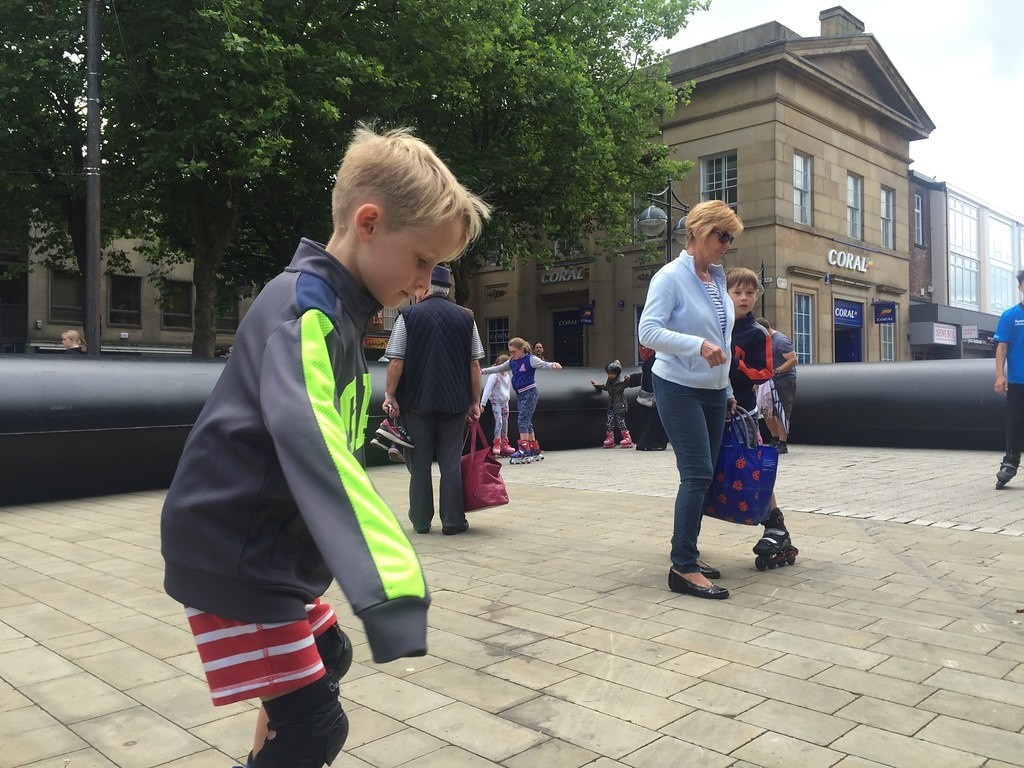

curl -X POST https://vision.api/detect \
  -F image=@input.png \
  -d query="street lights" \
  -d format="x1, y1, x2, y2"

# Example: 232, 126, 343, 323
637, 176, 690, 263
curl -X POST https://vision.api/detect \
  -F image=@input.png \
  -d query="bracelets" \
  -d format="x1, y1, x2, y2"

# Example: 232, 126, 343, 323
385, 393, 396, 398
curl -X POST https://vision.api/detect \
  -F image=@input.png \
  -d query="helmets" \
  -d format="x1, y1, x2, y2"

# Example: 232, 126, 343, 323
605, 362, 622, 377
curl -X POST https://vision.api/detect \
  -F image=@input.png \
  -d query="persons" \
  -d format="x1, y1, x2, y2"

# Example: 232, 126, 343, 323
993, 270, 1024, 490
220, 345, 233, 359
533, 342, 545, 361
638, 199, 743, 598
480, 338, 562, 464
591, 346, 667, 450
382, 266, 485, 535
480, 354, 515, 458
757, 318, 797, 454
62, 330, 87, 354
161, 127, 484, 768
726, 267, 799, 571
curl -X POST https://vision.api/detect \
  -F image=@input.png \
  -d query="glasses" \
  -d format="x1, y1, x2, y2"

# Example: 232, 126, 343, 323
534, 346, 543, 350
712, 229, 735, 246
509, 348, 521, 356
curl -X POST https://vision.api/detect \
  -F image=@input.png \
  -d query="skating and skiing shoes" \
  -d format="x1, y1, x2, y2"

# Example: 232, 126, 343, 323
499, 436, 516, 457
996, 454, 1021, 488
509, 438, 532, 464
491, 436, 502, 459
528, 440, 544, 461
752, 509, 799, 571
619, 429, 633, 448
603, 430, 616, 448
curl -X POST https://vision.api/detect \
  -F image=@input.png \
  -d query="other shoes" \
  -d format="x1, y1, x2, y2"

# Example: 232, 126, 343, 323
648, 446, 664, 450
774, 443, 789, 454
667, 567, 730, 600
416, 526, 430, 533
442, 517, 468, 534
695, 559, 720, 579
768, 438, 780, 448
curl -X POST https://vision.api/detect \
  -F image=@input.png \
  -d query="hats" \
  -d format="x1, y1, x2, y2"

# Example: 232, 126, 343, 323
431, 265, 453, 289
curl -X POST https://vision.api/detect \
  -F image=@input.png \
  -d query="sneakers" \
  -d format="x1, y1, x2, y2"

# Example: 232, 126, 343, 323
376, 416, 415, 449
370, 435, 392, 452
387, 441, 406, 463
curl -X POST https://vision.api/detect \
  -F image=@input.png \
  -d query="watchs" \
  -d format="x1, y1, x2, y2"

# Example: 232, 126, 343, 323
774, 369, 778, 375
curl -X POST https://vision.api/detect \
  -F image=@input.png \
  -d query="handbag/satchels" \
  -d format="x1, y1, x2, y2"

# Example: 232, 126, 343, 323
636, 389, 656, 407
460, 419, 509, 513
701, 404, 779, 525
